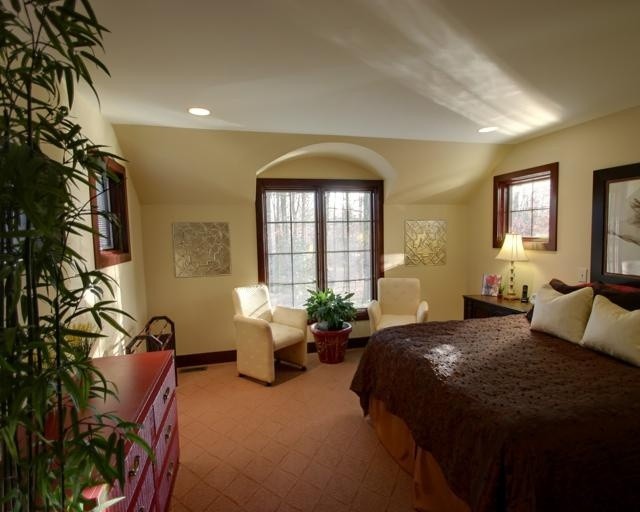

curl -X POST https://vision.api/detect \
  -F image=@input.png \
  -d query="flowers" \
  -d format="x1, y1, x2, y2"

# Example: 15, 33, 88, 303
66, 323, 101, 359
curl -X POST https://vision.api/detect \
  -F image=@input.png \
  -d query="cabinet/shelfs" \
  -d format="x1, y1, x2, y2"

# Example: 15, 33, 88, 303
124, 316, 176, 391
0, 348, 180, 512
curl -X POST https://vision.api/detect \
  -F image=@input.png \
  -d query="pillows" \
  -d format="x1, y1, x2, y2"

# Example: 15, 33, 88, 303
531, 283, 595, 344
596, 289, 640, 311
582, 295, 640, 368
525, 279, 582, 320
577, 283, 638, 292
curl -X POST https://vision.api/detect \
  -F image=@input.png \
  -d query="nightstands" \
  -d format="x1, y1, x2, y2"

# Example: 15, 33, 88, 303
464, 295, 533, 319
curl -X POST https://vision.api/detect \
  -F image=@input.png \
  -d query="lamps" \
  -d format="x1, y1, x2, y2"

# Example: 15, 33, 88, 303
496, 234, 528, 300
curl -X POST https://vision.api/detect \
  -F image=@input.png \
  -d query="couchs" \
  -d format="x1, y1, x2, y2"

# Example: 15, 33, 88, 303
233, 284, 308, 385
367, 277, 428, 334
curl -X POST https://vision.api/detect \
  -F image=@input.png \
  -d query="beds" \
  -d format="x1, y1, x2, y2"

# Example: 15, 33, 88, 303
349, 278, 640, 512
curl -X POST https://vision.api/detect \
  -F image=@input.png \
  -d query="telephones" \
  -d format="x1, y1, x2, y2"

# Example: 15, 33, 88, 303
521, 285, 529, 303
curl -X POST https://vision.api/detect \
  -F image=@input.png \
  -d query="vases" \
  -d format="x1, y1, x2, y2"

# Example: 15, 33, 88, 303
81, 357, 95, 388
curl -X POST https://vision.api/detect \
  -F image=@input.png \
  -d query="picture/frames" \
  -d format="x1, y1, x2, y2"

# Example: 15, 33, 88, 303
590, 162, 640, 287
482, 273, 502, 297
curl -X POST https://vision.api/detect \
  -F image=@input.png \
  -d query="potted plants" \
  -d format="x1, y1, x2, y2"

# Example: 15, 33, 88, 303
303, 289, 357, 363
497, 283, 505, 298
